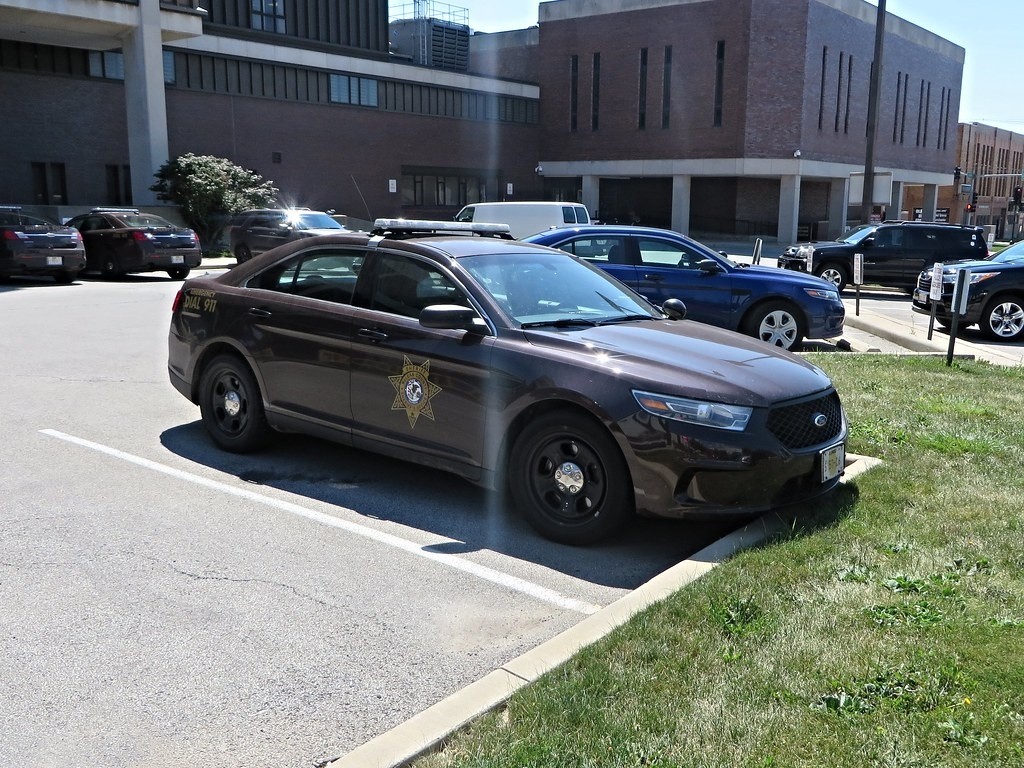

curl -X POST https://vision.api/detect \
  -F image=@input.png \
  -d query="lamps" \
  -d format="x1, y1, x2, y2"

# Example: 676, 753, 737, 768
506, 180, 513, 195
388, 177, 397, 193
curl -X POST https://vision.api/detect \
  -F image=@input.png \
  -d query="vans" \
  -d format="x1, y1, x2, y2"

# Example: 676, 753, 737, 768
450, 202, 594, 256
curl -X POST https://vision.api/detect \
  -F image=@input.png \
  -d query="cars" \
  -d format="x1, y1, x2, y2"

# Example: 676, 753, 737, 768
474, 225, 846, 353
0, 206, 87, 284
230, 210, 355, 270
911, 239, 1024, 344
168, 219, 849, 545
62, 208, 202, 282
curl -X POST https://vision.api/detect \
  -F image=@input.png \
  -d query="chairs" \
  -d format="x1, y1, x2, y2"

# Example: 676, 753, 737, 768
99, 217, 118, 229
7, 215, 29, 225
606, 243, 626, 265
878, 229, 892, 245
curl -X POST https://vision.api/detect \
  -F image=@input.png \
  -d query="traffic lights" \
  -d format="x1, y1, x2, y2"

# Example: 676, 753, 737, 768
1013, 187, 1022, 205
953, 166, 961, 180
967, 204, 971, 209
971, 192, 978, 212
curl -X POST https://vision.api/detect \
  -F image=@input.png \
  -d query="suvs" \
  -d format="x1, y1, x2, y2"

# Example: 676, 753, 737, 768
778, 220, 990, 294
